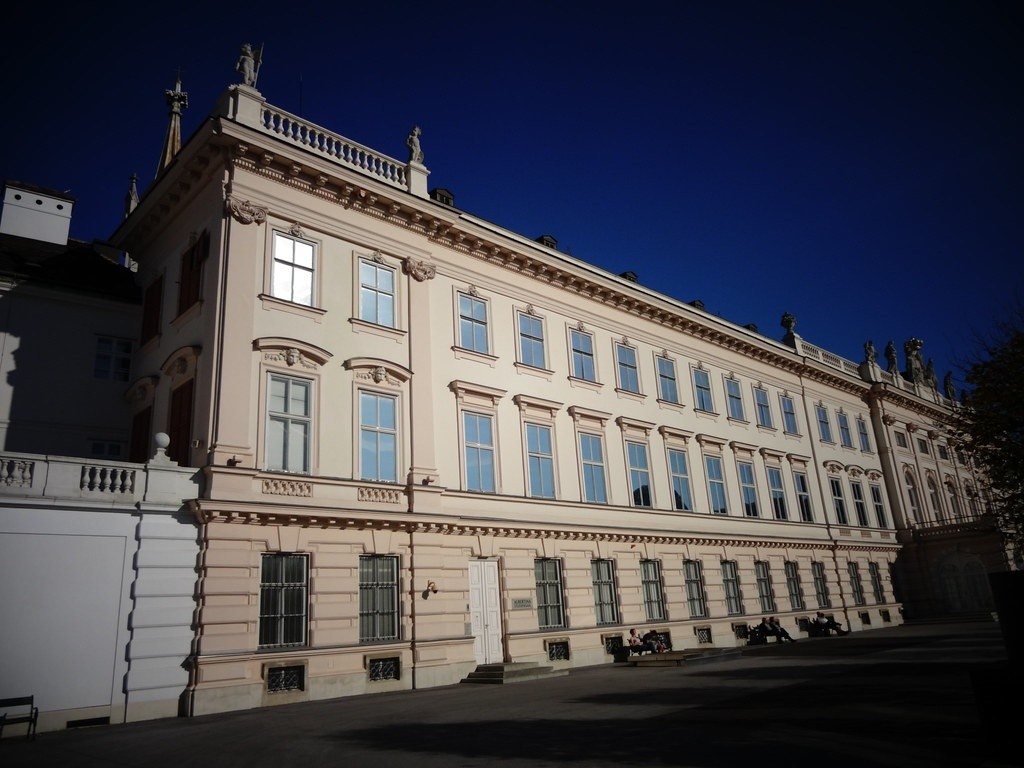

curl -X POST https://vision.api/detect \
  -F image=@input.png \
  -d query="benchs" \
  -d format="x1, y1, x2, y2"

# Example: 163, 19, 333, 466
627, 637, 663, 656
0, 695, 39, 740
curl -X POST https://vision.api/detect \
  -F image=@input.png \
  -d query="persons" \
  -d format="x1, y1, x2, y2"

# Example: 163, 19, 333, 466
816, 612, 849, 636
407, 128, 424, 163
759, 617, 797, 644
235, 41, 254, 84
884, 340, 897, 373
630, 629, 663, 653
864, 340, 876, 361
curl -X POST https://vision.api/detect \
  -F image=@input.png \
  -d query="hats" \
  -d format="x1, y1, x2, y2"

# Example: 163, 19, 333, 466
650, 629, 657, 636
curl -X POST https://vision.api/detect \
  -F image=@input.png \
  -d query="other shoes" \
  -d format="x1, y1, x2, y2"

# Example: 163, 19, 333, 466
779, 641, 784, 644
790, 640, 796, 643
